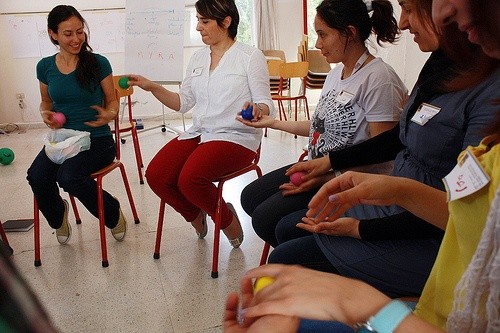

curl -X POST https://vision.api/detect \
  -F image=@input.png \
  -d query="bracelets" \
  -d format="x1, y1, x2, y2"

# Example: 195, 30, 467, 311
108, 108, 117, 120
356, 298, 411, 333
333, 169, 342, 177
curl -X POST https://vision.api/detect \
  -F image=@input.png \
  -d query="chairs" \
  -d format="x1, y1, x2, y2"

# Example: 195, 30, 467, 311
254, 144, 308, 286
113, 76, 144, 186
262, 39, 332, 139
33, 89, 140, 266
153, 143, 262, 278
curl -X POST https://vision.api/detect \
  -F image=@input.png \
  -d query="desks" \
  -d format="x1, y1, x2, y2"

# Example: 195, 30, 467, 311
120, 80, 187, 145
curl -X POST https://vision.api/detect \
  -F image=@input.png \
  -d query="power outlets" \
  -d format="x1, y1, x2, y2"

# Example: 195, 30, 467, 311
15, 92, 25, 99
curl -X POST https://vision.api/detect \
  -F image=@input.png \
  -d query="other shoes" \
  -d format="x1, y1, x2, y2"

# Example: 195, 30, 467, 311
195, 208, 209, 240
223, 202, 244, 249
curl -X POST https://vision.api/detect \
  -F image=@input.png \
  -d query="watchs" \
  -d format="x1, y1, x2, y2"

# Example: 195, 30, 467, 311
254, 103, 263, 110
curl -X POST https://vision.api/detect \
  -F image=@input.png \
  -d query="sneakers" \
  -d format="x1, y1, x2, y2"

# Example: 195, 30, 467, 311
111, 196, 127, 243
56, 199, 72, 245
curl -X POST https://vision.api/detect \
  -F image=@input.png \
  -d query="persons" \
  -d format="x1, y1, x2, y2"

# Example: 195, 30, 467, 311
224, 0, 500, 333
26, 4, 126, 245
121, 0, 275, 248
